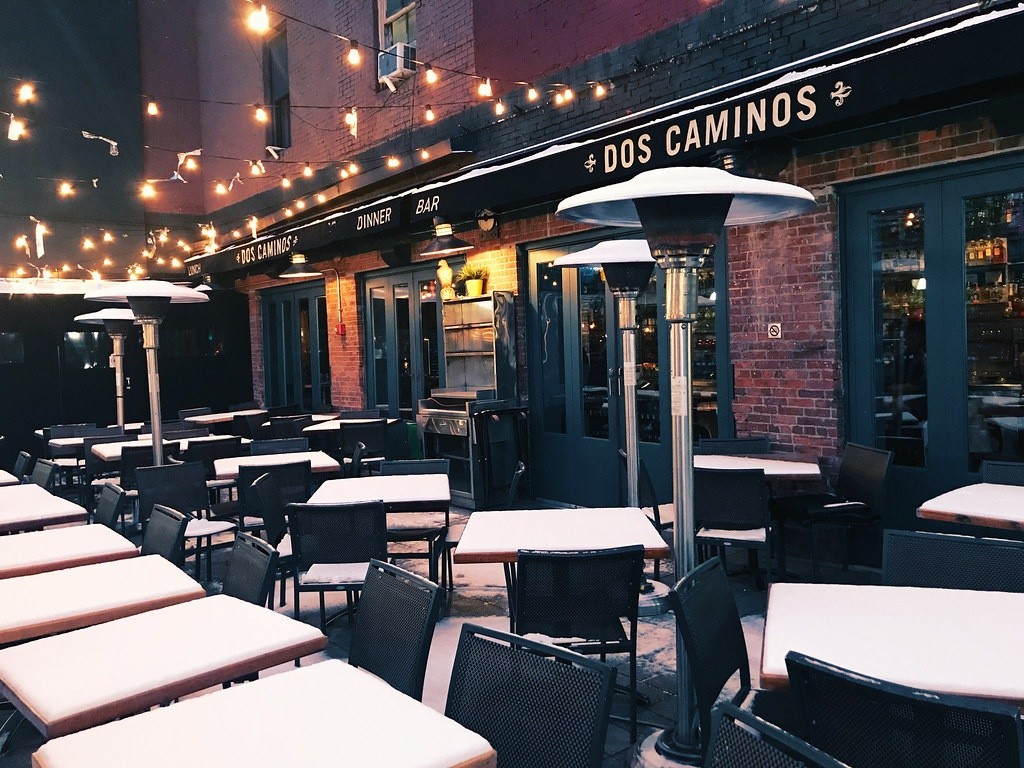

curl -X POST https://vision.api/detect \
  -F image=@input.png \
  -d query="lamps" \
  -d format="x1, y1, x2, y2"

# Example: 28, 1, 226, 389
278, 253, 322, 278
419, 216, 475, 256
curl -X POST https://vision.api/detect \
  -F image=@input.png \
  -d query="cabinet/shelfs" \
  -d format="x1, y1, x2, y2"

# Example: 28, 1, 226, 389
417, 289, 517, 513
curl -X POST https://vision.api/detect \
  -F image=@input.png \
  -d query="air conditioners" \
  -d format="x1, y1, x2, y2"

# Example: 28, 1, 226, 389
377, 41, 417, 85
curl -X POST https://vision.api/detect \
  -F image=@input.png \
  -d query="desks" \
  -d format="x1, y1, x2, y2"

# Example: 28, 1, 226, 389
0, 408, 1024, 768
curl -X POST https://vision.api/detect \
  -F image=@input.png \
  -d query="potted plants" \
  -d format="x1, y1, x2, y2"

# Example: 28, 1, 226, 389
455, 263, 492, 297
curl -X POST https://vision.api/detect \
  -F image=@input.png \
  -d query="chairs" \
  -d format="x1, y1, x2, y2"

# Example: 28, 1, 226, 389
11, 400, 1024, 768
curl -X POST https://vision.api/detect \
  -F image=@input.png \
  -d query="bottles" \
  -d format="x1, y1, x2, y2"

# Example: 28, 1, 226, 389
966, 277, 1024, 305
881, 248, 925, 272
966, 237, 1004, 263
